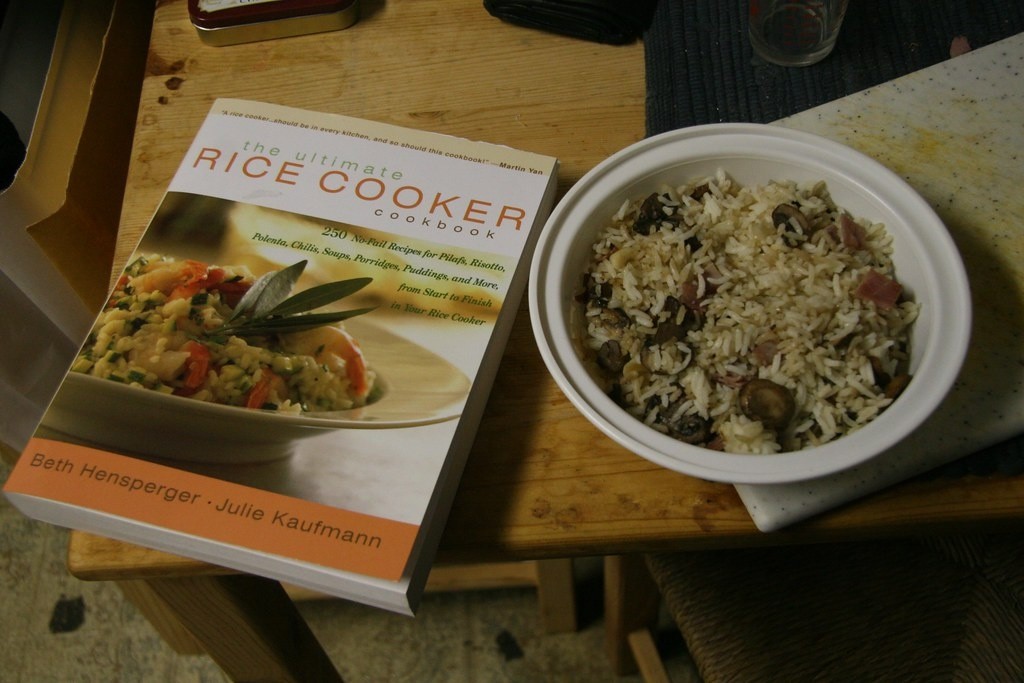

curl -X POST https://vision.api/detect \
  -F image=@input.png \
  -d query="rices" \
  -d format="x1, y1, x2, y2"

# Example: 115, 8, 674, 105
571, 166, 921, 453
70, 254, 378, 416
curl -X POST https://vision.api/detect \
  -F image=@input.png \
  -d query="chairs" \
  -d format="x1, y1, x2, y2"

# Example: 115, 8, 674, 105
606, 513, 1023, 683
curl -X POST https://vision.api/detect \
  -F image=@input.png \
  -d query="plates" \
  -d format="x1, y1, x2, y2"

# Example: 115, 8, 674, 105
40, 297, 473, 464
528, 124, 972, 483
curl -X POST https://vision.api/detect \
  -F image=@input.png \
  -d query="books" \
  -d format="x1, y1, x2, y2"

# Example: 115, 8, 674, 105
1, 98, 561, 618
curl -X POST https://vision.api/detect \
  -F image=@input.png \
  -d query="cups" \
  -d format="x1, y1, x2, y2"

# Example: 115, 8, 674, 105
747, 0, 848, 66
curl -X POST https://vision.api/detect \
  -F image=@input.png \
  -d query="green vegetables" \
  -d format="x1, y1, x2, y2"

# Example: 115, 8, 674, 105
206, 259, 380, 339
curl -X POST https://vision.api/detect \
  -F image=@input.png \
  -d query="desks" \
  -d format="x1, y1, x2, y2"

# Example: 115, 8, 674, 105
68, 0, 1023, 682
